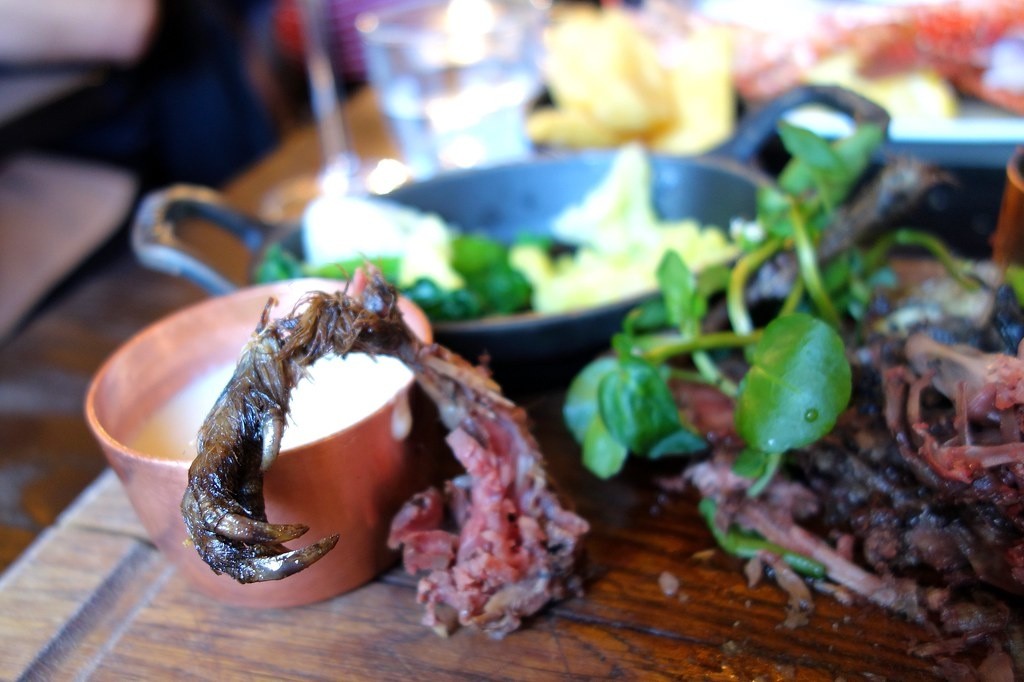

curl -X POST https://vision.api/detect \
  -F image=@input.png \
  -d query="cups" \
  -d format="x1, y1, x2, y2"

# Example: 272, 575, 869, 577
354, 0, 559, 185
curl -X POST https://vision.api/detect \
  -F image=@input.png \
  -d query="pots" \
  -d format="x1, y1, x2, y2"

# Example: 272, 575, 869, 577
126, 77, 892, 445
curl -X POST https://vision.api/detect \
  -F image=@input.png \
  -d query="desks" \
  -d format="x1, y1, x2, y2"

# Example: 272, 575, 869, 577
0, 256, 1024, 682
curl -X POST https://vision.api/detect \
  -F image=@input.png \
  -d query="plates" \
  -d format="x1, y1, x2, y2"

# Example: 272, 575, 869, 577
778, 42, 1024, 175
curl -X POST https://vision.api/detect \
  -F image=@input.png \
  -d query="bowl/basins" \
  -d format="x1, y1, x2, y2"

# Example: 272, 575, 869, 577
88, 275, 433, 612
